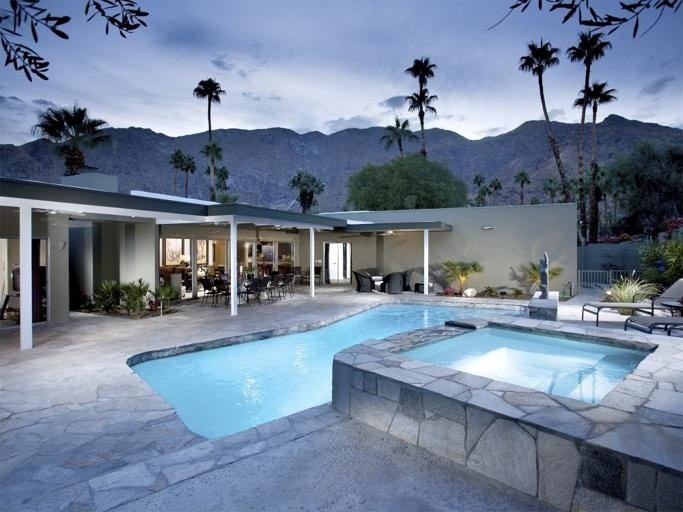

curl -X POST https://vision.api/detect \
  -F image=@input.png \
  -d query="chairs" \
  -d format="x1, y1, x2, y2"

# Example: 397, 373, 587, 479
582, 278, 683, 327
380, 272, 404, 294
201, 266, 321, 308
624, 315, 683, 336
353, 270, 372, 292
